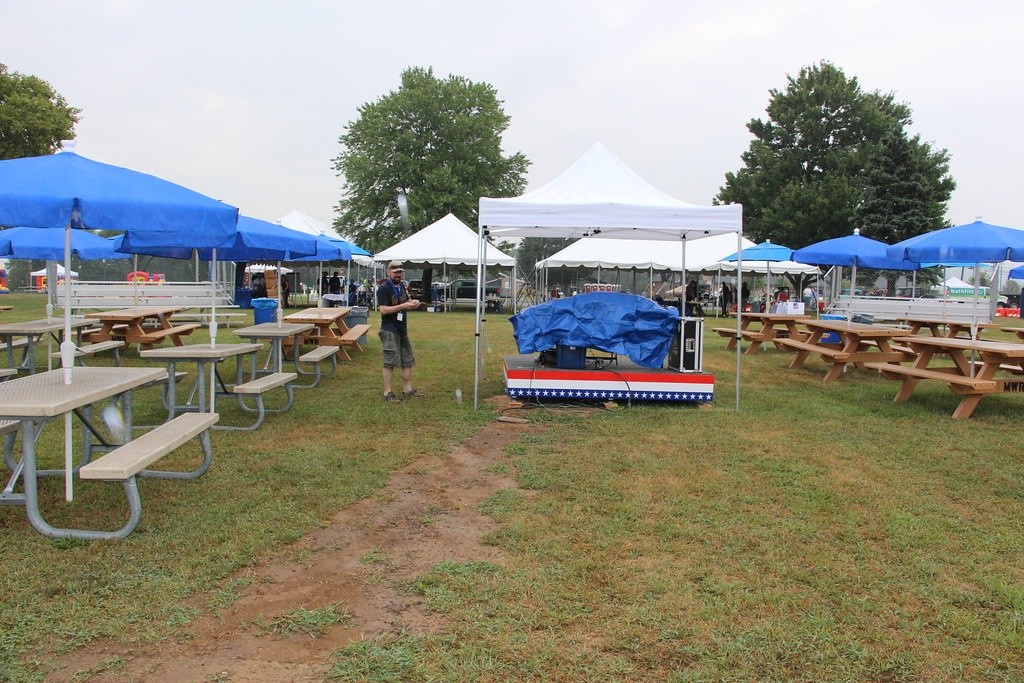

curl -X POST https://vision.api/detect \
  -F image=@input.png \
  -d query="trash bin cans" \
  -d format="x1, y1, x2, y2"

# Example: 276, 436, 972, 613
251, 298, 279, 325
322, 294, 344, 308
851, 313, 875, 325
345, 307, 369, 345
819, 313, 848, 343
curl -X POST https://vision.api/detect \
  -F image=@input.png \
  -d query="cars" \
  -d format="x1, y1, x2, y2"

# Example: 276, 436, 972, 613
326, 275, 501, 302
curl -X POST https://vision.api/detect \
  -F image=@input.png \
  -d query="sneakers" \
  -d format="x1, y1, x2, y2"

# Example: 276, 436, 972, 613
402, 389, 426, 399
383, 391, 400, 403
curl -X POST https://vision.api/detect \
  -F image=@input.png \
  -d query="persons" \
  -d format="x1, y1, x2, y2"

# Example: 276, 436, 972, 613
774, 285, 817, 310
254, 273, 268, 299
653, 294, 665, 305
861, 285, 910, 302
422, 260, 433, 304
494, 288, 505, 313
300, 282, 308, 294
281, 274, 290, 308
547, 288, 582, 300
317, 272, 408, 310
376, 261, 421, 401
685, 280, 750, 317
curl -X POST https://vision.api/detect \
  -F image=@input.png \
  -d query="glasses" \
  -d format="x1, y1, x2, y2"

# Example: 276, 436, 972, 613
389, 265, 403, 269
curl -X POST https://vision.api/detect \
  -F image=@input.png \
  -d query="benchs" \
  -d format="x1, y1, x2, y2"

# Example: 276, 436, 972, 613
0, 324, 373, 539
825, 294, 993, 331
711, 327, 1015, 390
55, 281, 247, 328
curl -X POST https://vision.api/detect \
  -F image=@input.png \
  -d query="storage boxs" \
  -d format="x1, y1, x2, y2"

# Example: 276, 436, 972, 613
264, 269, 285, 308
667, 317, 704, 373
556, 345, 586, 369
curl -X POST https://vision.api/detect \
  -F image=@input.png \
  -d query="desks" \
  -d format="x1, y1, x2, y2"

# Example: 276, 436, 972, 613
0, 305, 363, 540
725, 313, 1024, 420
485, 295, 504, 313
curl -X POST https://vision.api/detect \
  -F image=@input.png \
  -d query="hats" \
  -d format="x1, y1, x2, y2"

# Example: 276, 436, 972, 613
387, 261, 405, 272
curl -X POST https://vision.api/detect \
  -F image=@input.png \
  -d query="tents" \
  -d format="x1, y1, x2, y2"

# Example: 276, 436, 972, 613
930, 276, 986, 298
374, 213, 517, 314
532, 233, 822, 320
244, 211, 386, 283
474, 142, 743, 413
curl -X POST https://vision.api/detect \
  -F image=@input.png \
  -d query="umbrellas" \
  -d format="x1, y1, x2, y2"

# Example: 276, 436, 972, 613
790, 228, 921, 372
284, 234, 376, 335
717, 239, 793, 314
886, 216, 1024, 379
0, 151, 238, 504
0, 214, 317, 413
1007, 265, 1024, 279
